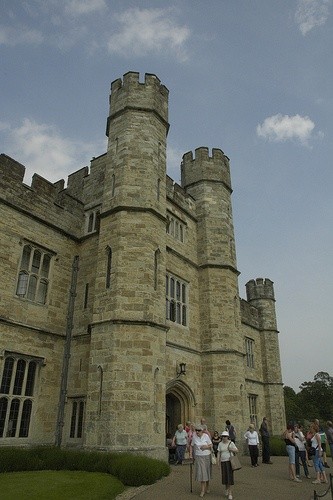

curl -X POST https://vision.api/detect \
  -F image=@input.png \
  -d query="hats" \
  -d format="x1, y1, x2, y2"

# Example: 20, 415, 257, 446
220, 430, 229, 436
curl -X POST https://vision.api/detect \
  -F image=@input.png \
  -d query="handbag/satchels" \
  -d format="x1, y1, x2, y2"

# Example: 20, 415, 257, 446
307, 444, 319, 456
228, 441, 241, 470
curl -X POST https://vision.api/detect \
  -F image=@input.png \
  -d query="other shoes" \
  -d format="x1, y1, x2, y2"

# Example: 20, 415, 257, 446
252, 463, 259, 467
289, 478, 302, 483
306, 475, 311, 478
311, 479, 328, 484
308, 464, 313, 467
296, 475, 300, 478
205, 488, 211, 493
200, 491, 205, 497
321, 462, 330, 468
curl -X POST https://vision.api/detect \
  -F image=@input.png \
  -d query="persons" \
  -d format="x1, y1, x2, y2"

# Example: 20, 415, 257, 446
166, 418, 211, 465
225, 419, 236, 444
259, 417, 273, 464
284, 418, 333, 485
211, 430, 222, 458
216, 430, 239, 500
188, 424, 214, 497
244, 424, 260, 468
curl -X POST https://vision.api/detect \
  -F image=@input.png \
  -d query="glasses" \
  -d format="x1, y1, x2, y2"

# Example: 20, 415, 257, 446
195, 429, 202, 432
221, 435, 228, 437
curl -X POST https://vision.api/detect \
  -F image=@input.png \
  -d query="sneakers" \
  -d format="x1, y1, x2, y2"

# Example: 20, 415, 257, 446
225, 490, 233, 499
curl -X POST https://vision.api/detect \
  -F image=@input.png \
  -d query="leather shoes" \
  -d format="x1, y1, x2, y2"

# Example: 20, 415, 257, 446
262, 461, 273, 464
